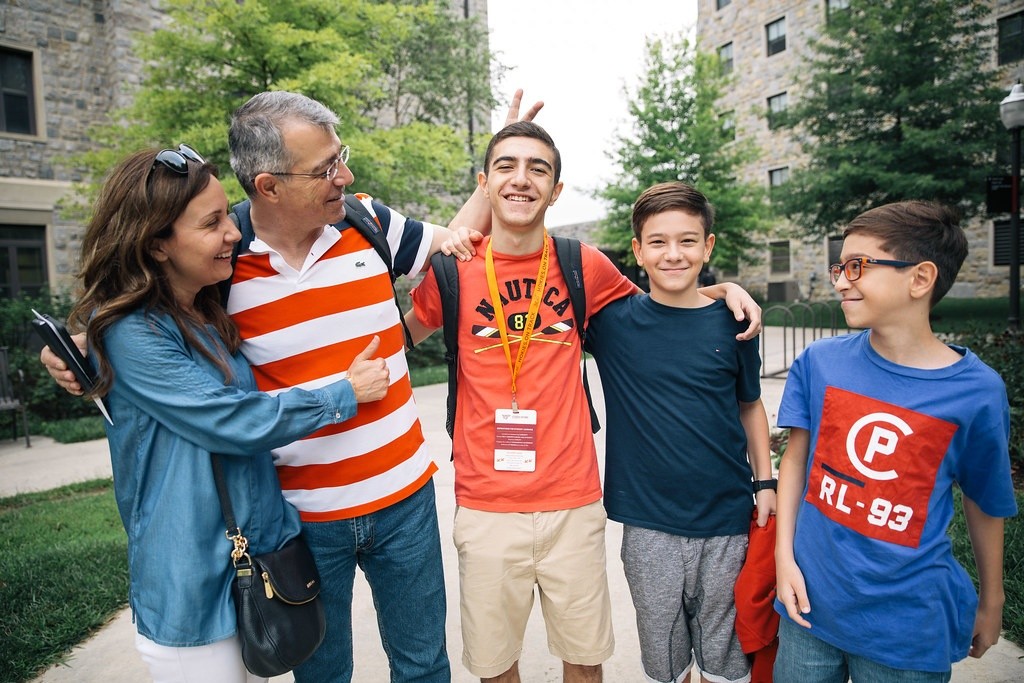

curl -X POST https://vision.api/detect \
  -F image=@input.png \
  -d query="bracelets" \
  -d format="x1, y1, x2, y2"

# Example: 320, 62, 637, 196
753, 479, 777, 494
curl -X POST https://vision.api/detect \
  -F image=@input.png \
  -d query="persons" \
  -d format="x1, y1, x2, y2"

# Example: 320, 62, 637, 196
774, 199, 1019, 683
40, 88, 544, 683
68, 144, 391, 682
441, 181, 777, 683
403, 119, 762, 683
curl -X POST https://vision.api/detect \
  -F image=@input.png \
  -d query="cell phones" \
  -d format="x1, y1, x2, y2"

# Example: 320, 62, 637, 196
32, 314, 103, 394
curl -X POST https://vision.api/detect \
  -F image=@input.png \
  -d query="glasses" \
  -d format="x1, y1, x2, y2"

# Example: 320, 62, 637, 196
830, 257, 920, 286
146, 143, 207, 189
252, 145, 350, 191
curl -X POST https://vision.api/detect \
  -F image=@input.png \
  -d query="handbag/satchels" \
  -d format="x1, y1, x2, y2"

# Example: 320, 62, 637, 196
231, 536, 326, 678
